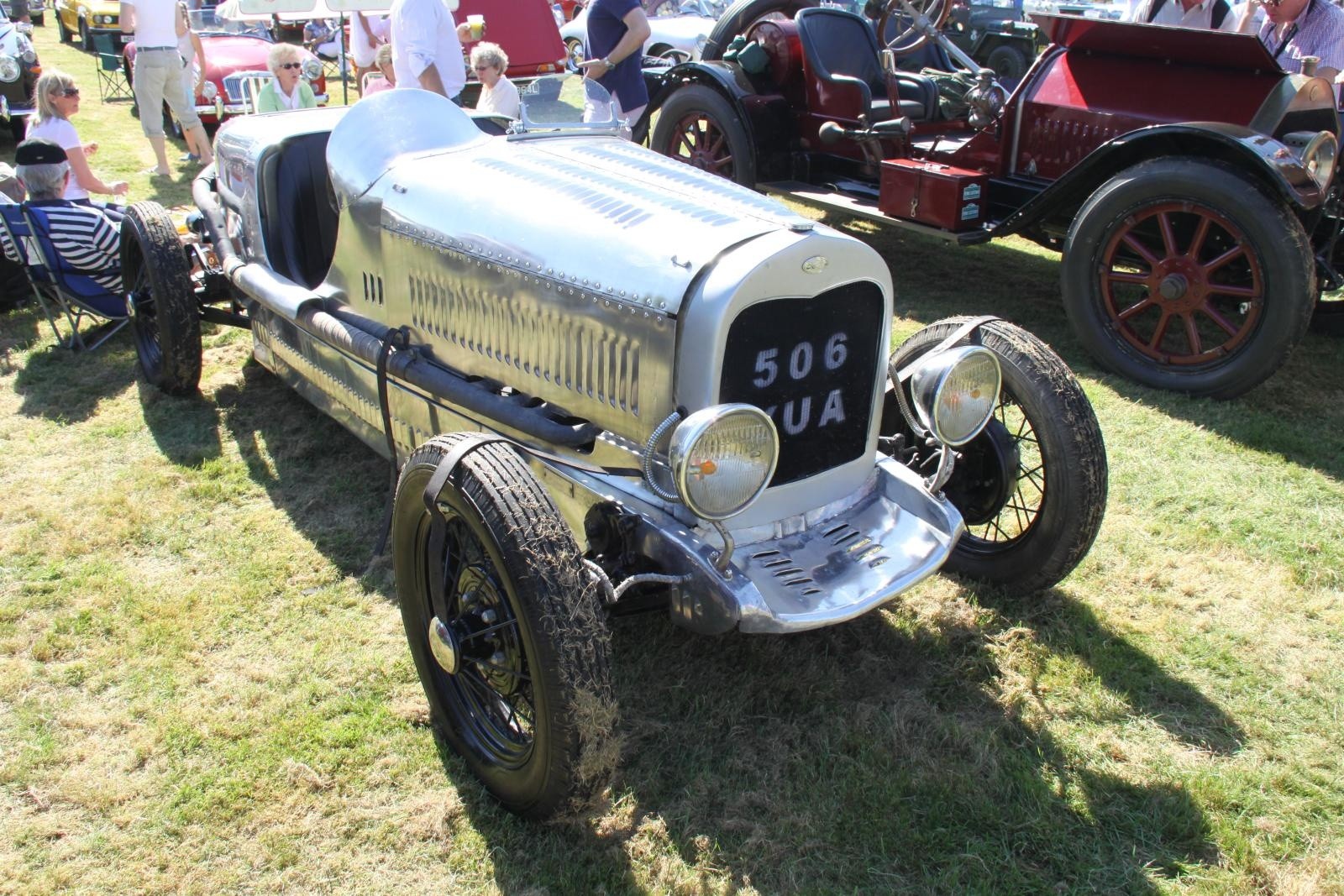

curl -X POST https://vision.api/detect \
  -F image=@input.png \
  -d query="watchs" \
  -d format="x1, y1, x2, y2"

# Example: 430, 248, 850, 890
604, 57, 615, 70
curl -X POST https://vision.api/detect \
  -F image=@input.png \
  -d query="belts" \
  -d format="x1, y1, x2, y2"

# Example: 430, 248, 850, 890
137, 47, 176, 52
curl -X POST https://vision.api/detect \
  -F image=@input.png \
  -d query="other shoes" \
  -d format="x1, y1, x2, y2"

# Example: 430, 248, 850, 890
180, 152, 201, 161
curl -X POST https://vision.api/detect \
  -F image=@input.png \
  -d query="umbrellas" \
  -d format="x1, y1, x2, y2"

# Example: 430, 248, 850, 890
215, 0, 393, 105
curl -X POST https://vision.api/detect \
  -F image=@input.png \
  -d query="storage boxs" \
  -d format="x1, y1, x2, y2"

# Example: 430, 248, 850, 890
879, 157, 989, 234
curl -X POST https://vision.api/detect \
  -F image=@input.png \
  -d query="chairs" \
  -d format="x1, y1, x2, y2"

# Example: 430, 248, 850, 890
91, 33, 135, 106
302, 42, 340, 79
0, 203, 133, 354
260, 131, 339, 292
795, 7, 939, 163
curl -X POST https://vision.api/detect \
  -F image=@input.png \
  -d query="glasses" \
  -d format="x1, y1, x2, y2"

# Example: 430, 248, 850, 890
1259, 0, 1283, 6
474, 65, 491, 71
279, 62, 300, 70
50, 88, 79, 99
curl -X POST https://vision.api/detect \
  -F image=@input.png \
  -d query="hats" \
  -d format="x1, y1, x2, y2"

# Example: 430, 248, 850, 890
15, 138, 68, 165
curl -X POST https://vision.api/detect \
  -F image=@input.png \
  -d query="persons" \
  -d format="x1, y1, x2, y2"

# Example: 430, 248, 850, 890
470, 41, 520, 118
258, 44, 317, 113
305, 18, 351, 75
391, 0, 487, 106
1133, 0, 1344, 83
119, 0, 214, 177
0, 138, 213, 296
25, 73, 128, 221
350, 11, 395, 96
577, 0, 651, 140
0, 163, 26, 204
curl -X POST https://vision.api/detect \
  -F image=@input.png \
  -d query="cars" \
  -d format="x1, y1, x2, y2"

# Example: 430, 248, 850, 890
1, 0, 330, 141
554, 0, 1344, 401
117, 73, 1110, 823
